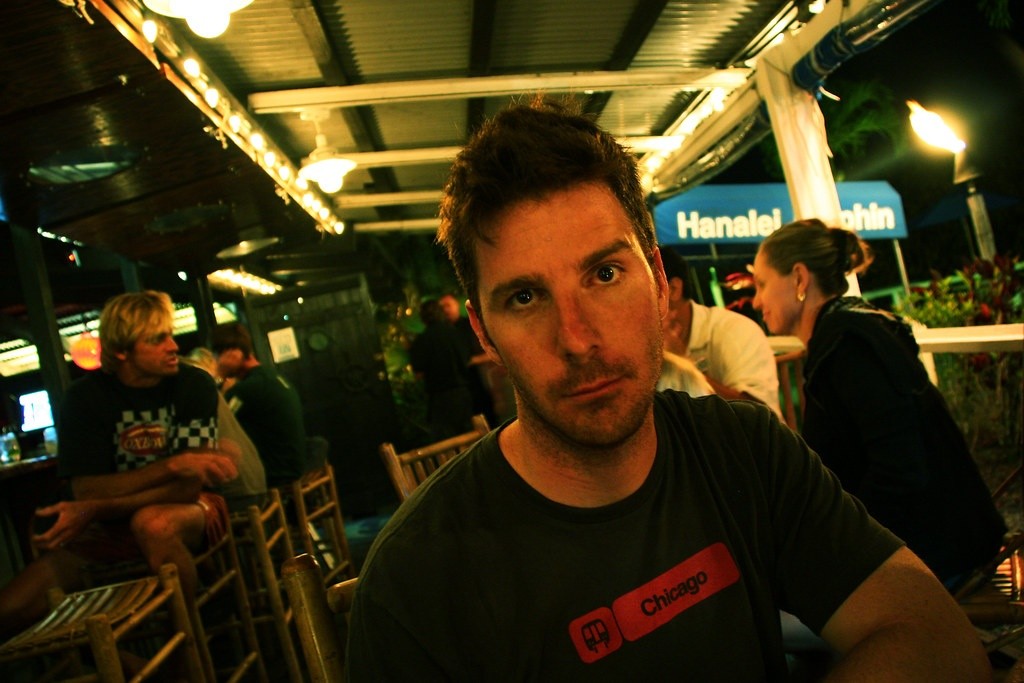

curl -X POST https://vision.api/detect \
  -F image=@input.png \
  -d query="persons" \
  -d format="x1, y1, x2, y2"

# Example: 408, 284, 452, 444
751, 217, 1010, 681
213, 389, 269, 515
657, 247, 785, 423
440, 295, 497, 430
0, 289, 242, 662
407, 300, 497, 450
656, 351, 717, 403
348, 105, 992, 683
211, 322, 311, 495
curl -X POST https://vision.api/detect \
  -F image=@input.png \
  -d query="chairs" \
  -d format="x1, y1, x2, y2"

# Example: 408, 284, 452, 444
378, 414, 490, 503
281, 553, 359, 683
951, 460, 1024, 654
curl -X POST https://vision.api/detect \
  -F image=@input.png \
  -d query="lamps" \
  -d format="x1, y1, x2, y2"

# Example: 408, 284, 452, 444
297, 109, 357, 194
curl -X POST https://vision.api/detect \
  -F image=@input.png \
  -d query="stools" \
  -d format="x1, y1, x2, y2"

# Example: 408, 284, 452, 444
211, 488, 304, 683
265, 464, 355, 580
95, 524, 269, 683
0, 563, 207, 683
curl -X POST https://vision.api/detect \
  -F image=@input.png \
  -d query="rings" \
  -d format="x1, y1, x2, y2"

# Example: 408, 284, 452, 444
59, 542, 65, 548
